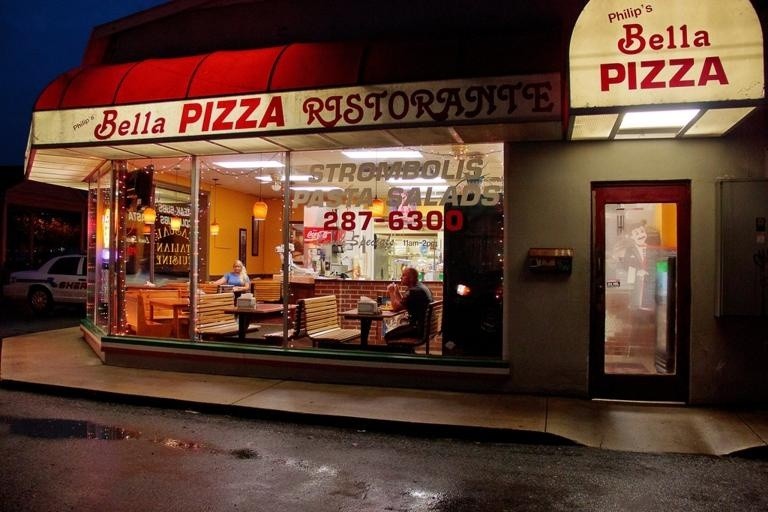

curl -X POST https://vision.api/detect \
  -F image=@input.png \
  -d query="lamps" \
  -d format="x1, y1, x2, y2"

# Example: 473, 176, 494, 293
252, 167, 268, 221
140, 164, 221, 235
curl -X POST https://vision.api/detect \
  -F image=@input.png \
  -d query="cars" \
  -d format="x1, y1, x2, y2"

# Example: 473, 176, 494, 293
3, 255, 87, 314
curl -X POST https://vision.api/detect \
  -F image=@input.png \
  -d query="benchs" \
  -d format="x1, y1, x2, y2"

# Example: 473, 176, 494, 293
386, 300, 443, 355
126, 279, 361, 348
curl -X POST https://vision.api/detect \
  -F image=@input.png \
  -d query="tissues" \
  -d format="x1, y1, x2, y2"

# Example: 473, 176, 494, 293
144, 281, 155, 288
358, 296, 377, 315
237, 293, 256, 309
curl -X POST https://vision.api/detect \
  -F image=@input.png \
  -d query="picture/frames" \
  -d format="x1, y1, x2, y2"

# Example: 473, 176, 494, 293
239, 216, 260, 268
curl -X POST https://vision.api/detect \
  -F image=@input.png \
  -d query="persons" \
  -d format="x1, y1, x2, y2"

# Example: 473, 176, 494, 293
384, 267, 434, 353
211, 259, 251, 321
